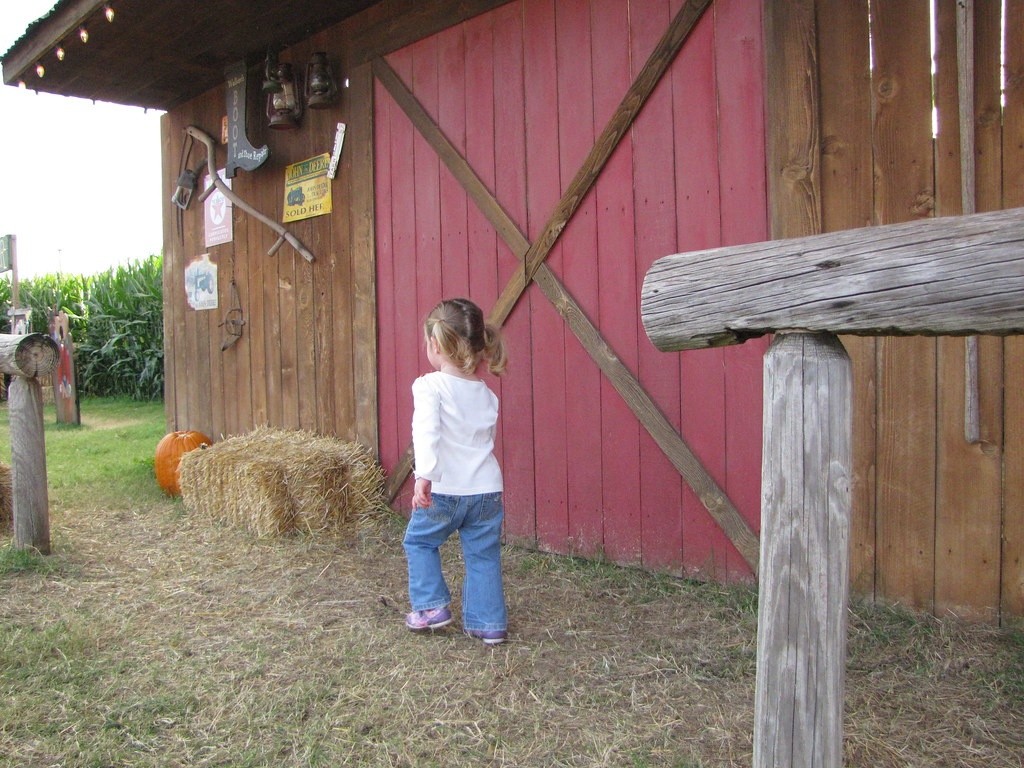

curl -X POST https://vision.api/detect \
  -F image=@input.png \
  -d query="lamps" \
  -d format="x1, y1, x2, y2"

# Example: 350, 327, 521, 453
263, 44, 303, 130
303, 52, 340, 110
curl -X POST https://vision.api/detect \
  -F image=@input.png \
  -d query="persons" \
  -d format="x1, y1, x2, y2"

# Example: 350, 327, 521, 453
402, 298, 507, 644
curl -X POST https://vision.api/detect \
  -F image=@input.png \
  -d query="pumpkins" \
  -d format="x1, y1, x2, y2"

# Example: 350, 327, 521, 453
154, 430, 212, 498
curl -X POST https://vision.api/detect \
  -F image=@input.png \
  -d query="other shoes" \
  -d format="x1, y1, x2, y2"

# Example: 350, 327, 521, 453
406, 607, 452, 629
465, 629, 505, 643
